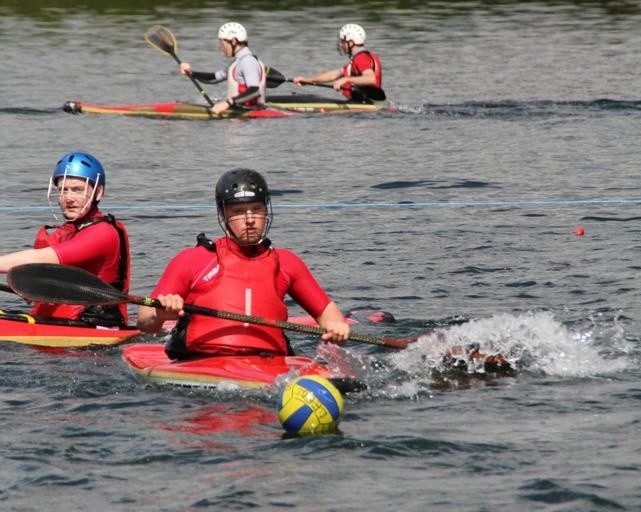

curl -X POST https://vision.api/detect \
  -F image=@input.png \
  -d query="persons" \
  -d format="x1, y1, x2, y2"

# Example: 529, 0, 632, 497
180, 22, 267, 115
293, 23, 382, 105
0, 151, 132, 330
137, 167, 351, 361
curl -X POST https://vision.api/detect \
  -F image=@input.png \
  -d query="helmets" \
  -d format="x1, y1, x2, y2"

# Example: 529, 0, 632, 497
215, 167, 275, 248
217, 21, 249, 44
46, 150, 107, 223
338, 23, 366, 55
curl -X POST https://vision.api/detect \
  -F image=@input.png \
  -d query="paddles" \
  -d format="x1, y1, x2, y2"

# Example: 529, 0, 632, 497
145, 25, 226, 119
6, 263, 508, 365
264, 66, 386, 101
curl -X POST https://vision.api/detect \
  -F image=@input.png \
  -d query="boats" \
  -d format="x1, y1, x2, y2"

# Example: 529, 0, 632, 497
0, 296, 400, 349
58, 88, 307, 123
254, 88, 421, 117
118, 336, 370, 404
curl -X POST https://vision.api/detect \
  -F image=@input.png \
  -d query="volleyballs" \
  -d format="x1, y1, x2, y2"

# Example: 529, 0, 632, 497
276, 375, 344, 435
